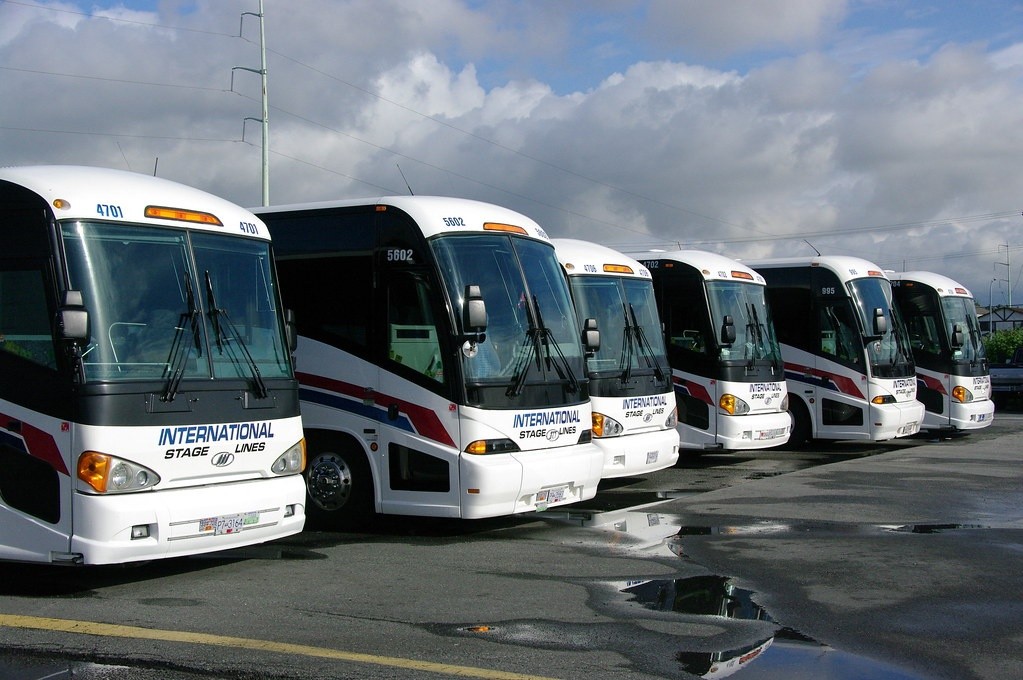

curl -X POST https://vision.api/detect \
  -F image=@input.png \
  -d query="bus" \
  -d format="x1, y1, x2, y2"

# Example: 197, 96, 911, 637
736, 255, 995, 450
244, 193, 792, 531
0, 164, 307, 568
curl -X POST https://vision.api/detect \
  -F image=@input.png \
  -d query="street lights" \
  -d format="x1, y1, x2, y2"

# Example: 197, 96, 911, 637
989, 278, 997, 341
